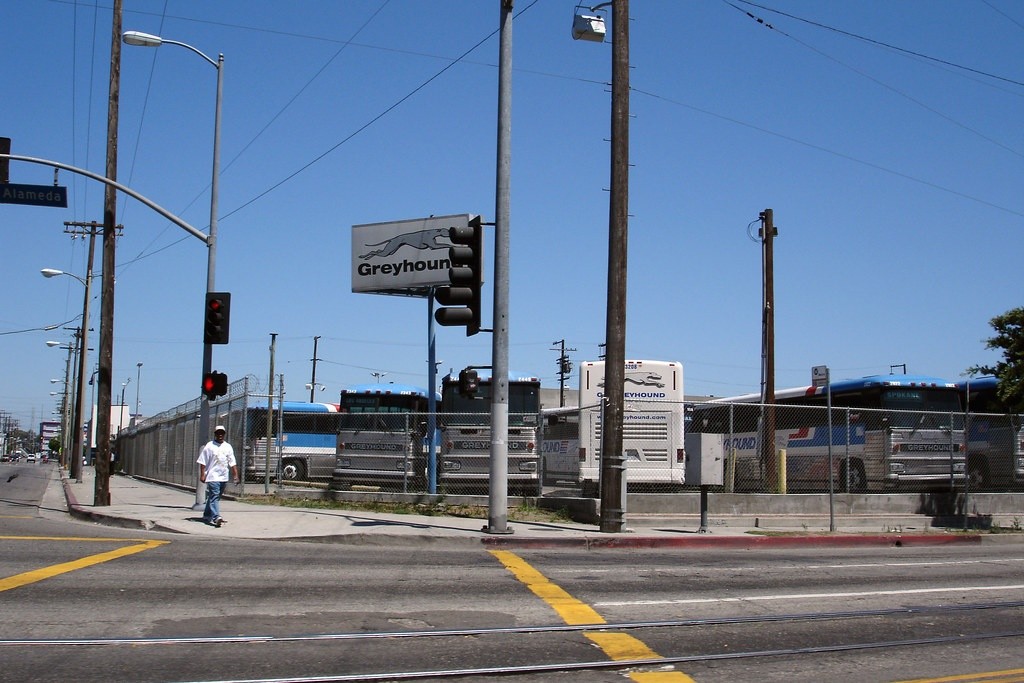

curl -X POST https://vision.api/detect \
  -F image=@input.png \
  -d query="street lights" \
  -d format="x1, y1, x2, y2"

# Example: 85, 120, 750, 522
137, 362, 143, 417
122, 29, 224, 512
46, 340, 77, 475
50, 379, 74, 471
40, 268, 92, 483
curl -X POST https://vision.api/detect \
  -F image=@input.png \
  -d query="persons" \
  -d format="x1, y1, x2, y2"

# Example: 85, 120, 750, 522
196, 426, 239, 528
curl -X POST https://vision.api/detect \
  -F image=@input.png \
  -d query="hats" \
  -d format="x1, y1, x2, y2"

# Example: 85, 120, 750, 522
213, 425, 226, 433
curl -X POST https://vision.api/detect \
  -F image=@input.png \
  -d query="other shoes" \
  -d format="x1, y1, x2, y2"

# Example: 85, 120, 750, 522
215, 516, 224, 526
206, 517, 217, 525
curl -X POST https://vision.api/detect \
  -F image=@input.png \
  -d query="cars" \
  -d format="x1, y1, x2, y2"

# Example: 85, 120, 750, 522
27, 454, 36, 463
2, 450, 22, 462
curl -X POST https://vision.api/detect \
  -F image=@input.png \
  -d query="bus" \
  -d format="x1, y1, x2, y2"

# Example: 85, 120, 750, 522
958, 376, 1024, 492
246, 403, 340, 481
694, 375, 969, 493
441, 368, 543, 496
579, 360, 685, 486
333, 382, 441, 488
541, 406, 579, 486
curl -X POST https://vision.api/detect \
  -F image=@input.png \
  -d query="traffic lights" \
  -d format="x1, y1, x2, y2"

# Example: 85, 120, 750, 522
435, 226, 474, 326
203, 291, 232, 344
203, 370, 228, 400
